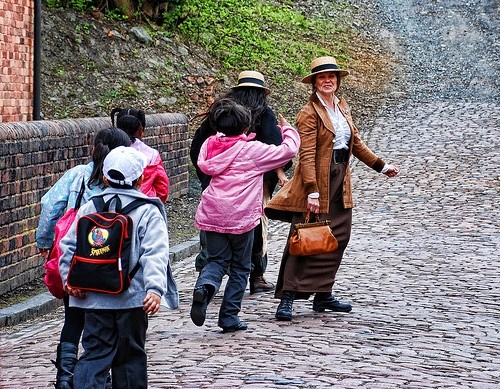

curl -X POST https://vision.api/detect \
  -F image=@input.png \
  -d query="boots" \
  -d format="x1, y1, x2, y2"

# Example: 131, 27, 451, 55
50, 342, 77, 389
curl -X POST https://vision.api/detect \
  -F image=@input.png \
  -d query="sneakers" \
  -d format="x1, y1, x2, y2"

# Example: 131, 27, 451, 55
250, 277, 277, 294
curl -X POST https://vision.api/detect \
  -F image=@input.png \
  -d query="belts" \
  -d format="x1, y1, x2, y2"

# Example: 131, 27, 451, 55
331, 150, 349, 165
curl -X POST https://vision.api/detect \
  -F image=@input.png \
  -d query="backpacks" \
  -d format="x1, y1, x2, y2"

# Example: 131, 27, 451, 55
67, 195, 148, 294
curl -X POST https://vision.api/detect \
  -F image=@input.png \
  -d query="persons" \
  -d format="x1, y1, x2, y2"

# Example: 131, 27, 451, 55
36, 127, 131, 389
58, 146, 179, 389
191, 71, 293, 292
190, 98, 301, 332
264, 56, 399, 319
112, 108, 168, 203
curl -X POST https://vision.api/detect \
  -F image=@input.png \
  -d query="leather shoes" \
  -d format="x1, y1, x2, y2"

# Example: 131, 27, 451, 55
313, 295, 352, 312
191, 289, 207, 327
275, 297, 293, 320
224, 323, 247, 332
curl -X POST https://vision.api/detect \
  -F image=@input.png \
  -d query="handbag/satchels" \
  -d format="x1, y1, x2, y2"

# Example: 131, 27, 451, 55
290, 208, 338, 256
45, 178, 86, 299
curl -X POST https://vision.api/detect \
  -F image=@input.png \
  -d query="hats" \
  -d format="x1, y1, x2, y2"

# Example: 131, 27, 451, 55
301, 56, 349, 84
230, 71, 271, 95
102, 145, 148, 186
